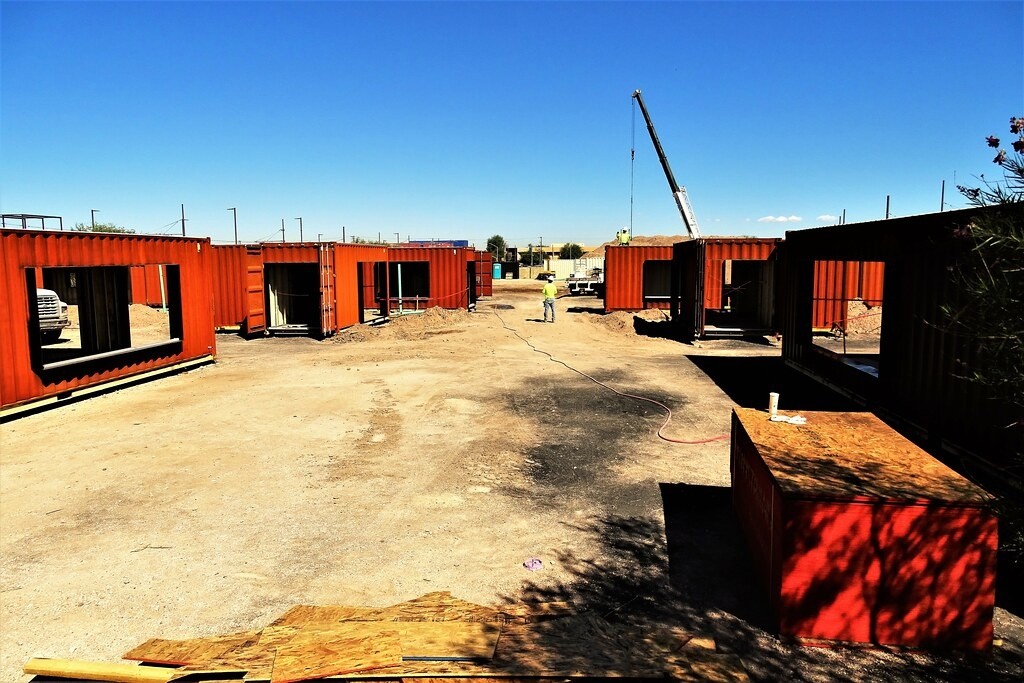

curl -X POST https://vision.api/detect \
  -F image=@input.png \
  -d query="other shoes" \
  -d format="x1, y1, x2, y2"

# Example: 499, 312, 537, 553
544, 318, 555, 323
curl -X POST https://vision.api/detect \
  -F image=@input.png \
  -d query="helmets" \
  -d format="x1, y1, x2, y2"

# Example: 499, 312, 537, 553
547, 276, 555, 281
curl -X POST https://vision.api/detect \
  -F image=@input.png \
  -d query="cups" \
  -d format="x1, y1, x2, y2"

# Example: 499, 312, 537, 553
769, 392, 779, 414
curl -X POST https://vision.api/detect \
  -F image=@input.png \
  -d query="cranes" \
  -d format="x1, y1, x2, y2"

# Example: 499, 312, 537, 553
625, 87, 704, 240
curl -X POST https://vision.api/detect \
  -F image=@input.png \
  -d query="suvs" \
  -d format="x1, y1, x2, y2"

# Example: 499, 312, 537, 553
36, 287, 68, 342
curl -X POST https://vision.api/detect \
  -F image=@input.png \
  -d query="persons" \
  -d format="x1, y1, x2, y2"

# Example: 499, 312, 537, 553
542, 275, 558, 322
616, 226, 632, 245
